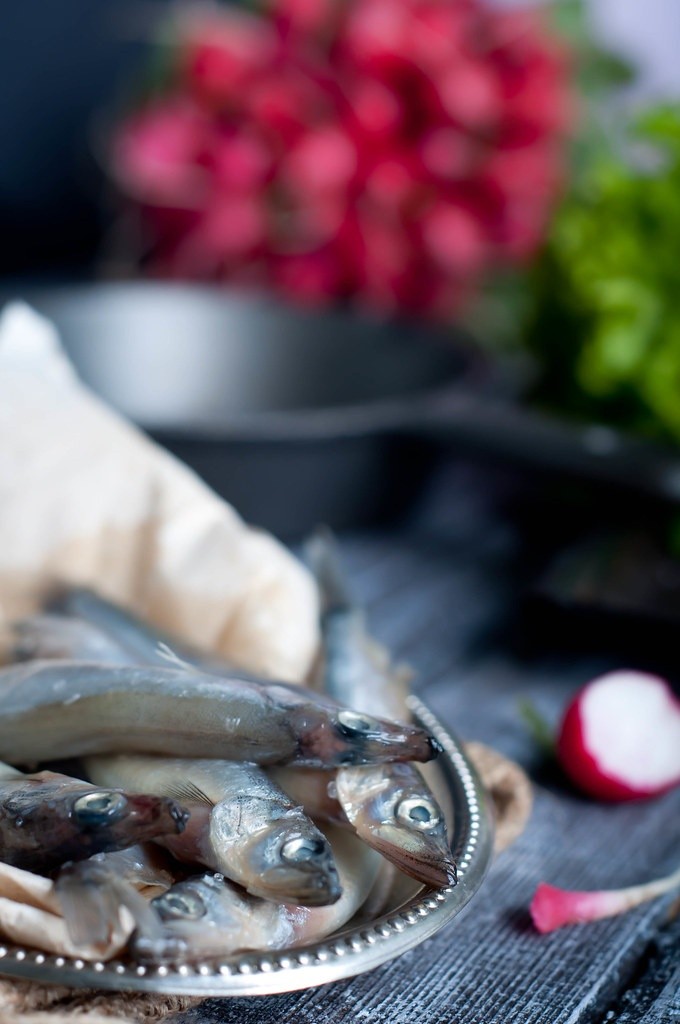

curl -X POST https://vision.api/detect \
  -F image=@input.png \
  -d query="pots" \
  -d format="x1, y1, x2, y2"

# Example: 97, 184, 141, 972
16, 282, 680, 542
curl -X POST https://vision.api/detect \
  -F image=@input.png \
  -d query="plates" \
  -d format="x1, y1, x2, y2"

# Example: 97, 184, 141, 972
0, 687, 498, 1000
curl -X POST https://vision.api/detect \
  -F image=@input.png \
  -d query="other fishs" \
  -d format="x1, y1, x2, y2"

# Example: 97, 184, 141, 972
0, 527, 462, 970
